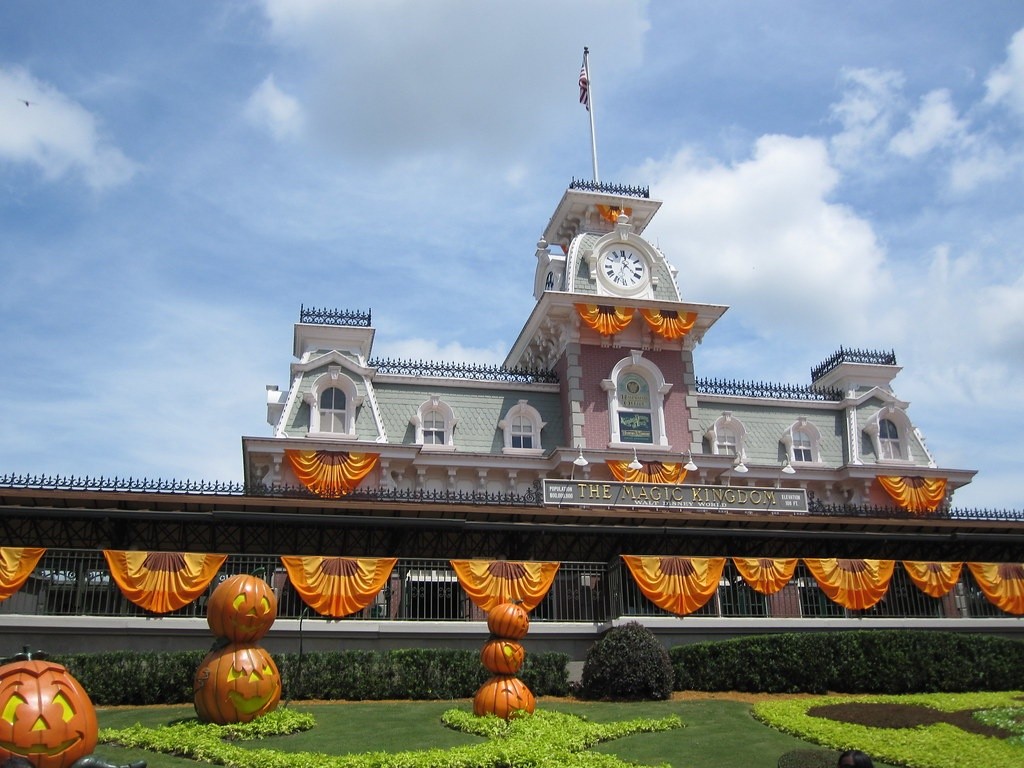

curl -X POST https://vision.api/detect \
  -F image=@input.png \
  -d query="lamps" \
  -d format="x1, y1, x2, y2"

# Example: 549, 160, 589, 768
776, 453, 796, 487
571, 444, 589, 480
625, 447, 644, 483
674, 449, 698, 484
727, 452, 749, 486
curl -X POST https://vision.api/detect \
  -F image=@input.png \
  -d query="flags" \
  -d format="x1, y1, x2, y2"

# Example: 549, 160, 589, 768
578, 51, 593, 111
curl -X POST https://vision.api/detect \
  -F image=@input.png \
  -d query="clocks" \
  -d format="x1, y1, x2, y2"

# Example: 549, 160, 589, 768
597, 243, 652, 299
